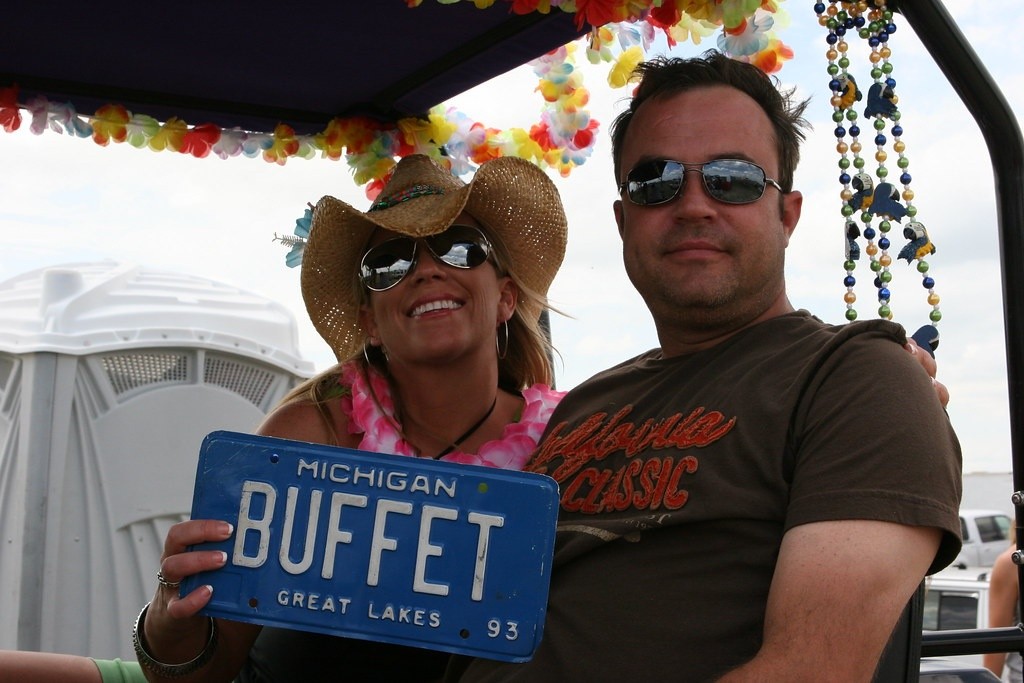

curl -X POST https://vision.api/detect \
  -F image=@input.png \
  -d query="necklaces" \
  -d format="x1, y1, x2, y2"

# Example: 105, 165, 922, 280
426, 387, 498, 463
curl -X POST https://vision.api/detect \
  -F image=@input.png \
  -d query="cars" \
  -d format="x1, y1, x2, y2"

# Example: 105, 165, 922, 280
916, 506, 1024, 683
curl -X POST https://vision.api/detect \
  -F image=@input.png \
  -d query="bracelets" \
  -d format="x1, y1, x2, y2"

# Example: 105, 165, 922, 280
133, 599, 217, 676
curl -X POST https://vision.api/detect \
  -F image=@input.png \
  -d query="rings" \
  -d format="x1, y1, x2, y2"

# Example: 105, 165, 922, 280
156, 571, 182, 589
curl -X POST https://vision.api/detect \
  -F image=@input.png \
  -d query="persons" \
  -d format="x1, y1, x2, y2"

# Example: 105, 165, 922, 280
522, 52, 965, 683
133, 152, 950, 682
982, 517, 1024, 683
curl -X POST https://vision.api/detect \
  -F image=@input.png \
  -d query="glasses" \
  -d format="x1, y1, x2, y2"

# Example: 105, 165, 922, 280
618, 156, 785, 207
355, 225, 504, 302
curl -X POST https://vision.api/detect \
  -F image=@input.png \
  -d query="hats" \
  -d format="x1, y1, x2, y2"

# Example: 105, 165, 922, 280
300, 155, 568, 364
